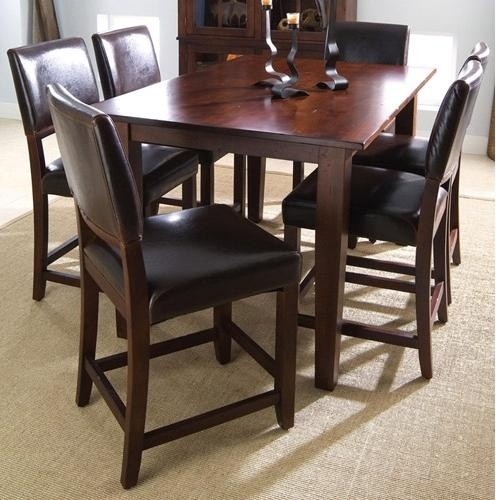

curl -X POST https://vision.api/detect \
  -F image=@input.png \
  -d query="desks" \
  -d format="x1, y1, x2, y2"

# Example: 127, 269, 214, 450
87, 57, 437, 394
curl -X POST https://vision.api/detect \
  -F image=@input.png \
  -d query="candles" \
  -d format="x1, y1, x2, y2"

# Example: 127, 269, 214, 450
261, 0, 272, 6
287, 12, 299, 24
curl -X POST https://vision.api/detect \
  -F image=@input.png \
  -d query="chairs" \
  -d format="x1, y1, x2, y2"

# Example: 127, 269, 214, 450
278, 58, 481, 377
89, 24, 248, 212
9, 38, 198, 301
290, 21, 408, 189
44, 81, 304, 490
349, 41, 488, 307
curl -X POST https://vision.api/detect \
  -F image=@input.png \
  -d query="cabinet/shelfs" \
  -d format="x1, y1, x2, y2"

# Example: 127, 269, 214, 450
176, 0, 359, 82
